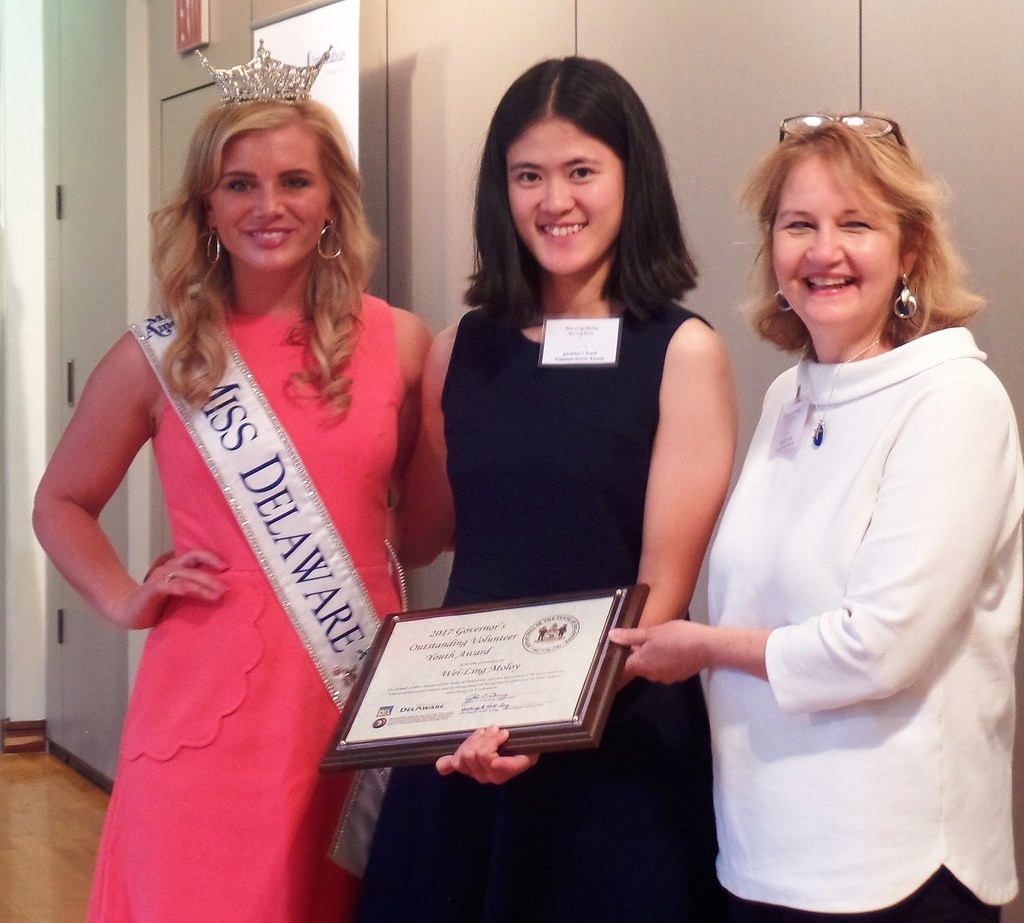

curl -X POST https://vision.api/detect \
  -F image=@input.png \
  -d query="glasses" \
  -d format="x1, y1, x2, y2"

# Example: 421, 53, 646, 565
778, 114, 907, 149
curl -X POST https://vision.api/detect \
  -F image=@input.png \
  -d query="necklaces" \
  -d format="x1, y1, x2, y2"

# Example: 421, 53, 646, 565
805, 338, 880, 446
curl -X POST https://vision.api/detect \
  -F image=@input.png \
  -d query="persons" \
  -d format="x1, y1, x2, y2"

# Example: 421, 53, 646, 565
352, 56, 738, 923
609, 110, 1024, 923
33, 40, 458, 923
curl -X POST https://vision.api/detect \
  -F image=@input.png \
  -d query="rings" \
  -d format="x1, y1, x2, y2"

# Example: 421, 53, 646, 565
163, 572, 175, 582
